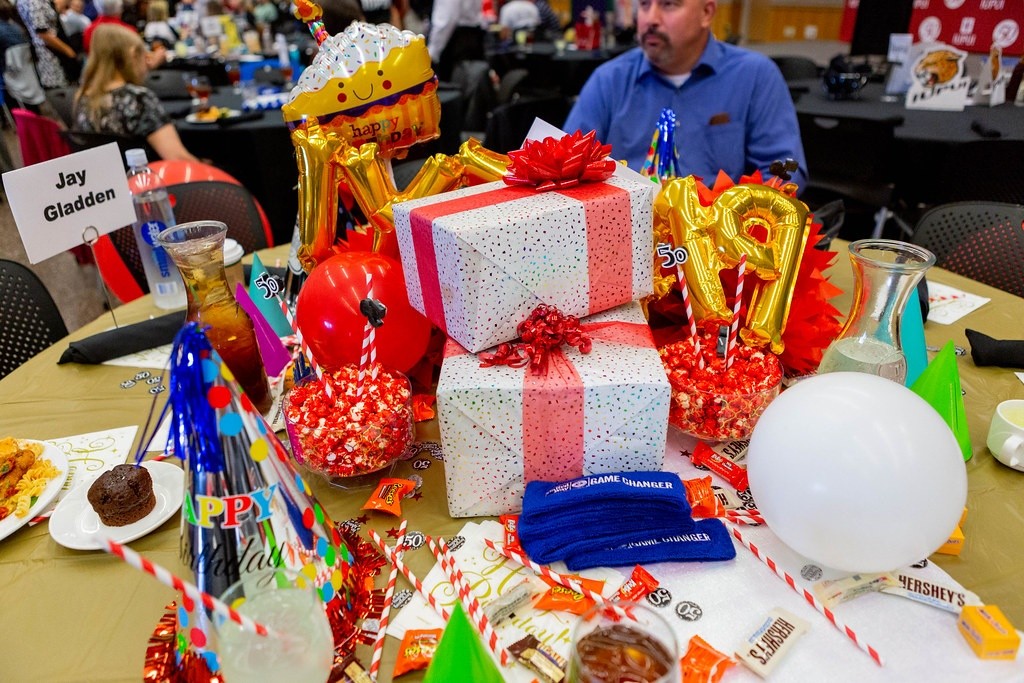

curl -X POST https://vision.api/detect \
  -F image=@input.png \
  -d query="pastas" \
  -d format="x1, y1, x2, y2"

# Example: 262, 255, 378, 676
2, 442, 62, 519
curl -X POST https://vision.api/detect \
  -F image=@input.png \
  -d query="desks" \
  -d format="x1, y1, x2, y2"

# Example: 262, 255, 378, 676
0, 242, 1024, 682
785, 80, 1024, 209
164, 87, 462, 246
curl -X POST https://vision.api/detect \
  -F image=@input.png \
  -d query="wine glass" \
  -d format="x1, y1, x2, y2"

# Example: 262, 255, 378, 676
175, 11, 198, 40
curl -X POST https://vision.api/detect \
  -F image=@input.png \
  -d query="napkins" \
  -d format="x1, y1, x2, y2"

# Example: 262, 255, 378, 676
917, 276, 930, 324
963, 329, 1024, 370
56, 310, 186, 365
242, 263, 286, 287
215, 113, 264, 128
971, 119, 1001, 138
169, 105, 192, 120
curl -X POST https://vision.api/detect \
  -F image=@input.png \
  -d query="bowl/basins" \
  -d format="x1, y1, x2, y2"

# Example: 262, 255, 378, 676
659, 358, 785, 442
281, 369, 416, 477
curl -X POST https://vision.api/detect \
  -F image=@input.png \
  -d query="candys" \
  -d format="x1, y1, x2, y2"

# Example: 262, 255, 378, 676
659, 335, 783, 441
279, 365, 436, 477
358, 480, 893, 683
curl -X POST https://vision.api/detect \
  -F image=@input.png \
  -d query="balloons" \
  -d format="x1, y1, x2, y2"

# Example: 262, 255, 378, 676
289, 123, 513, 276
654, 175, 813, 356
746, 370, 968, 575
297, 251, 432, 374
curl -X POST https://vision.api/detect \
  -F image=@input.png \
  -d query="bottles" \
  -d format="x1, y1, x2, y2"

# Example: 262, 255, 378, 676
816, 239, 936, 386
125, 148, 188, 309
156, 220, 272, 418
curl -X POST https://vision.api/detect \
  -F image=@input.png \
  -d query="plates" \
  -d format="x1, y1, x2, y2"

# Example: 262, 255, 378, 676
0, 439, 68, 541
185, 109, 240, 124
48, 462, 185, 550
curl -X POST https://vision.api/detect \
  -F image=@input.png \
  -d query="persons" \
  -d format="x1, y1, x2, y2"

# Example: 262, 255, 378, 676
0, 0, 632, 209
563, 0, 808, 196
72, 24, 205, 163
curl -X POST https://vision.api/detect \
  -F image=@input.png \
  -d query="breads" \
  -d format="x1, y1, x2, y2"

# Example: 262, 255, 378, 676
195, 105, 218, 122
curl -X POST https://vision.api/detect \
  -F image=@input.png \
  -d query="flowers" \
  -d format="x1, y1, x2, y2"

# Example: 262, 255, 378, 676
503, 128, 616, 191
478, 303, 592, 377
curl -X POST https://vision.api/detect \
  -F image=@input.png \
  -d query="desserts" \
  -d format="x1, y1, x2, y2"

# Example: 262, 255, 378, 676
86, 464, 156, 527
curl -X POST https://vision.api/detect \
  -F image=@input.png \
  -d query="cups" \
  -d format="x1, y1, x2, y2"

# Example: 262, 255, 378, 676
211, 567, 335, 683
226, 62, 241, 96
188, 77, 212, 121
200, 15, 222, 44
987, 399, 1024, 472
241, 31, 261, 52
221, 238, 244, 298
823, 71, 861, 101
237, 55, 263, 82
281, 62, 296, 89
516, 30, 567, 57
564, 599, 679, 683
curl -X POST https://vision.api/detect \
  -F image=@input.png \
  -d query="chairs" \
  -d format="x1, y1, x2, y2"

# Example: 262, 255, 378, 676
770, 56, 822, 82
796, 106, 1024, 297
0, 61, 540, 382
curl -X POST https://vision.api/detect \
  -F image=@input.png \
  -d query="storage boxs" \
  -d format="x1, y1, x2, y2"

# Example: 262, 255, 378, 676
435, 299, 671, 518
392, 175, 653, 354
934, 507, 968, 556
957, 605, 1020, 660
